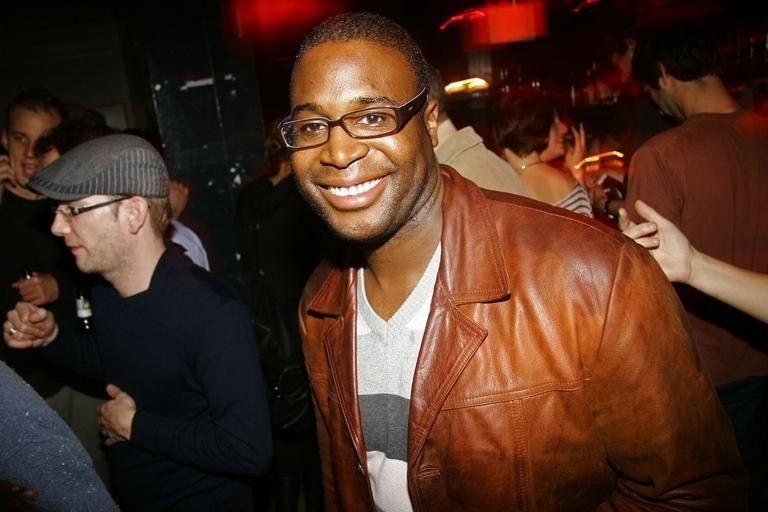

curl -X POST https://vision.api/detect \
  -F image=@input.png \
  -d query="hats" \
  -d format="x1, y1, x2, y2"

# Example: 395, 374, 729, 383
25, 135, 168, 202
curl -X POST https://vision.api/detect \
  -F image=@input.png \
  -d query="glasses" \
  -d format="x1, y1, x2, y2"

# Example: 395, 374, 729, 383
276, 88, 427, 150
60, 193, 137, 222
